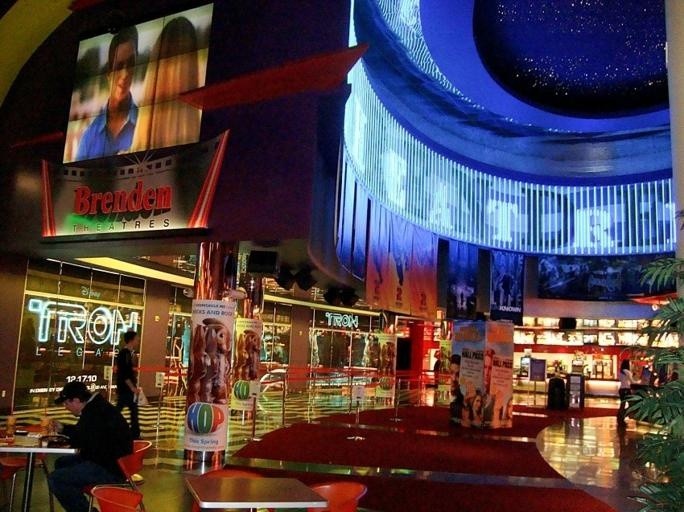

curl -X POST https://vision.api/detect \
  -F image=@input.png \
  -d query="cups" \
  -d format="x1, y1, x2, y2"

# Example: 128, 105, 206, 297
40, 419, 48, 434
6, 416, 16, 433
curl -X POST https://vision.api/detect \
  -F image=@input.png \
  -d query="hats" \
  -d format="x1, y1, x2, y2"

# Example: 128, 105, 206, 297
52, 380, 92, 406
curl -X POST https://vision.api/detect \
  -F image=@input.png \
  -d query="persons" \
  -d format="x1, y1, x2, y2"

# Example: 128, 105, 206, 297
615, 425, 641, 494
481, 346, 506, 429
448, 353, 475, 427
460, 290, 464, 307
500, 395, 512, 429
472, 388, 482, 427
114, 330, 144, 440
72, 23, 139, 162
539, 258, 674, 299
128, 16, 200, 155
616, 358, 642, 426
45, 380, 133, 512
497, 270, 515, 308
669, 371, 678, 383
